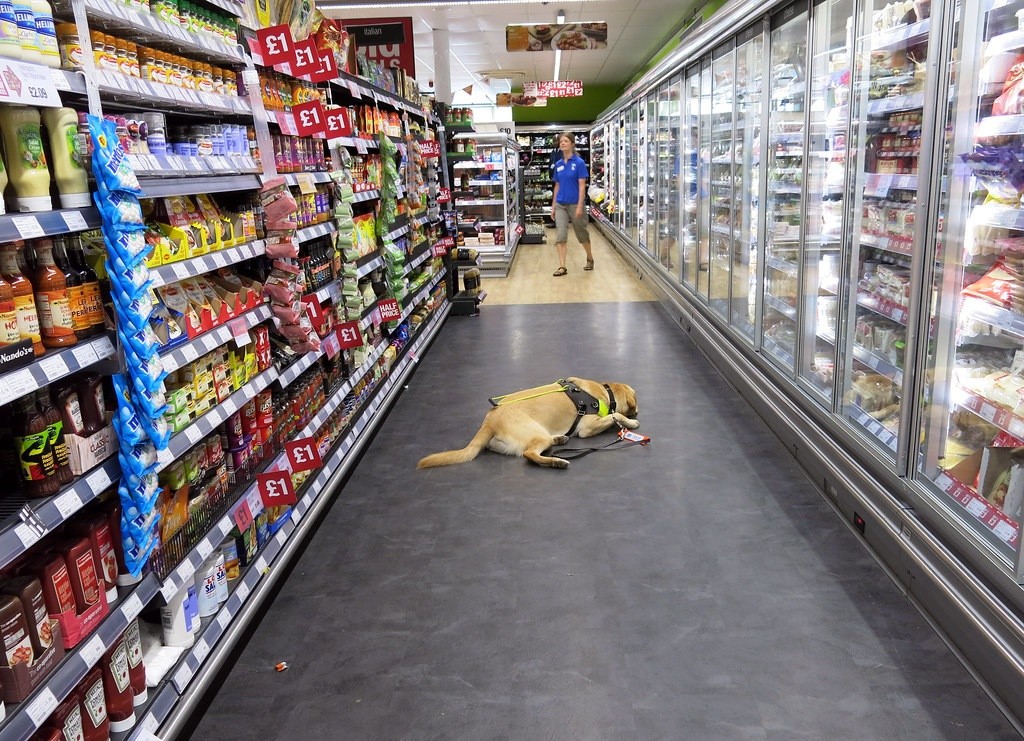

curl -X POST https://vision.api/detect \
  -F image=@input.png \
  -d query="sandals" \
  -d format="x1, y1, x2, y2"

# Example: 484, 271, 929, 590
700, 263, 708, 272
585, 259, 594, 270
661, 254, 674, 268
554, 267, 568, 276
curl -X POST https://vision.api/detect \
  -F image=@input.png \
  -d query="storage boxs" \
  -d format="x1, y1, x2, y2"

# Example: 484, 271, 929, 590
166, 328, 272, 430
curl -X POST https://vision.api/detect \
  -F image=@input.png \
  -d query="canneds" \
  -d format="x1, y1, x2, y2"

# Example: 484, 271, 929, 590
461, 174, 469, 191
447, 138, 476, 152
446, 108, 472, 123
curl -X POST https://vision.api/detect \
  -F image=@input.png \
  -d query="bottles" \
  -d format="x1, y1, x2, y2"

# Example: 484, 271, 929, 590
259, 68, 321, 116
292, 240, 333, 295
271, 129, 327, 173
35, 387, 74, 483
288, 183, 332, 230
10, 392, 58, 496
56, 0, 244, 95
0, 506, 148, 741
0, 232, 107, 357
52, 367, 108, 439
270, 356, 388, 460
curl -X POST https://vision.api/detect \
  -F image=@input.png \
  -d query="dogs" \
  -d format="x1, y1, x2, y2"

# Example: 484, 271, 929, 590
416, 377, 641, 471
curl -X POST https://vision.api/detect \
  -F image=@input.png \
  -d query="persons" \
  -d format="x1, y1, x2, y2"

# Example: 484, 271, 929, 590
550, 133, 593, 277
546, 134, 561, 229
659, 128, 713, 272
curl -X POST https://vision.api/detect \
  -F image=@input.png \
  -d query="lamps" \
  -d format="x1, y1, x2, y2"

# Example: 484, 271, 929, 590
553, 9, 566, 82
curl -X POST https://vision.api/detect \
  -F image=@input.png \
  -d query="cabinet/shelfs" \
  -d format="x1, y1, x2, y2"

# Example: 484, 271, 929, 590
519, 168, 548, 243
446, 122, 487, 317
515, 131, 591, 223
0, 0, 453, 741
453, 133, 522, 279
